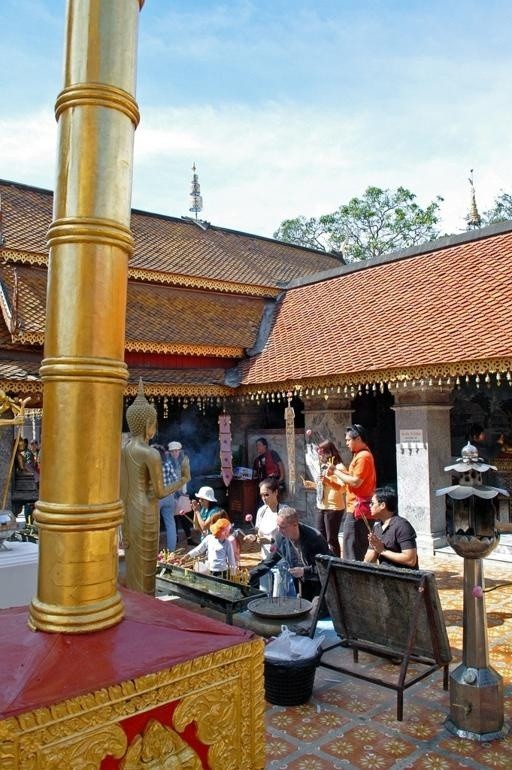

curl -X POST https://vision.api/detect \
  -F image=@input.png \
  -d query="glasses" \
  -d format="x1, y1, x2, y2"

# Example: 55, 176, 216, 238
261, 494, 269, 496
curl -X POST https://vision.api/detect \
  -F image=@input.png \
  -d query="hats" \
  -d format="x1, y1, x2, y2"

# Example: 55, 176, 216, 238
195, 486, 217, 502
168, 441, 182, 450
210, 519, 232, 540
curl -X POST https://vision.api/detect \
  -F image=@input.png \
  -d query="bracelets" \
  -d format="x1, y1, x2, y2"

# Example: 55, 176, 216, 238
269, 538, 275, 544
253, 535, 257, 542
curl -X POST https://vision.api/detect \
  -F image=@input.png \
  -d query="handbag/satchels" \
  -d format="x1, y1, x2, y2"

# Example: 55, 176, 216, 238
354, 496, 374, 520
174, 495, 192, 515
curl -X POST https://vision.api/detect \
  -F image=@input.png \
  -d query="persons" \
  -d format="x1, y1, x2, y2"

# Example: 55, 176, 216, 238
302, 441, 347, 558
164, 442, 198, 547
191, 485, 227, 543
464, 422, 507, 490
152, 443, 179, 552
243, 478, 289, 560
324, 423, 377, 561
121, 375, 191, 595
363, 485, 418, 571
252, 437, 286, 498
9, 437, 41, 543
248, 508, 335, 619
210, 509, 242, 563
177, 519, 235, 582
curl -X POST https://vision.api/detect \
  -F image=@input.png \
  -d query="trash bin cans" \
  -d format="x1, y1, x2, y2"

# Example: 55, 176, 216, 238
264, 634, 322, 706
